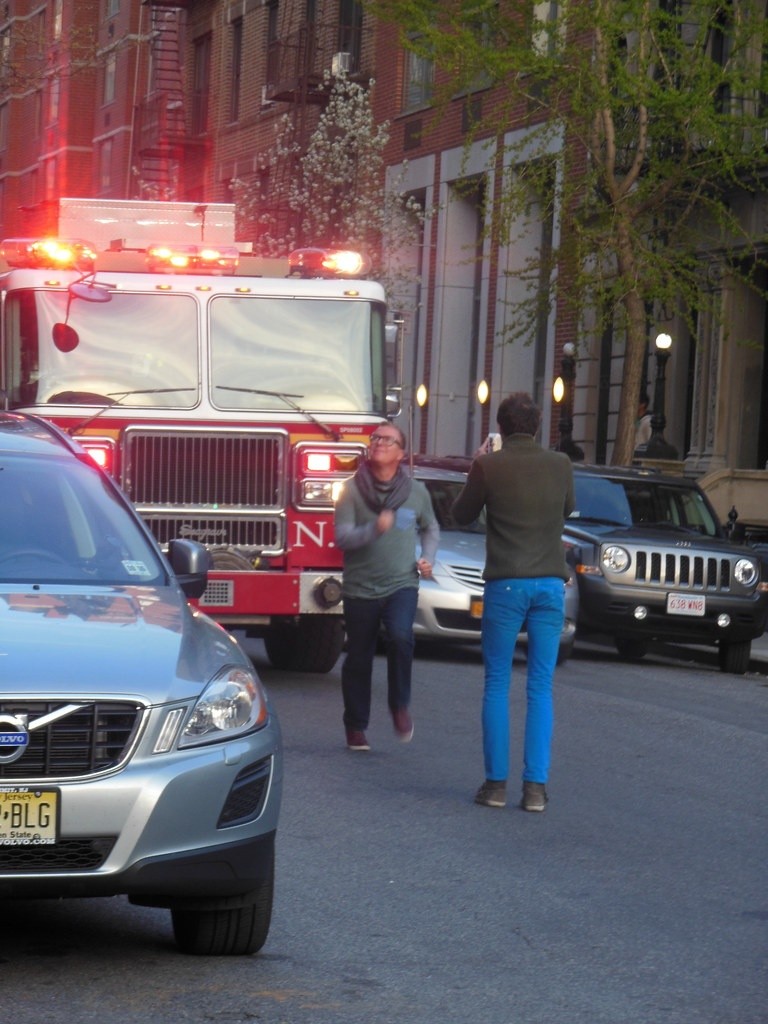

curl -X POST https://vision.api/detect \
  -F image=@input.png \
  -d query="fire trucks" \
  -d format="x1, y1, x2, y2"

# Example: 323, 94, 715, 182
0, 197, 404, 674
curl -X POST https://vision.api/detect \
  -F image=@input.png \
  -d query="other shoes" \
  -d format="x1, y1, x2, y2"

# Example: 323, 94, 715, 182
391, 707, 414, 740
521, 781, 548, 811
475, 780, 506, 807
345, 728, 371, 751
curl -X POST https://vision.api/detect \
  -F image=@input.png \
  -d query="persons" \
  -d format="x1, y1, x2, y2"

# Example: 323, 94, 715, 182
453, 391, 576, 811
632, 393, 655, 449
334, 421, 439, 749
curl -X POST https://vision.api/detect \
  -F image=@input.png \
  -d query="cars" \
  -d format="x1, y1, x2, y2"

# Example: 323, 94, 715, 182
561, 465, 768, 674
401, 464, 580, 665
0, 410, 284, 958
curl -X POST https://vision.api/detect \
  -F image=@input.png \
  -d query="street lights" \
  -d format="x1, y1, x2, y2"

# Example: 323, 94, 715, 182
634, 332, 678, 460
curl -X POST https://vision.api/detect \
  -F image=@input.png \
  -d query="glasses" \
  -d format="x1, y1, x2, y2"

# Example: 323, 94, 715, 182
369, 434, 403, 449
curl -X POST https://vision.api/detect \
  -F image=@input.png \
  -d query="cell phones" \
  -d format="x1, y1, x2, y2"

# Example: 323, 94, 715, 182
488, 432, 502, 453
